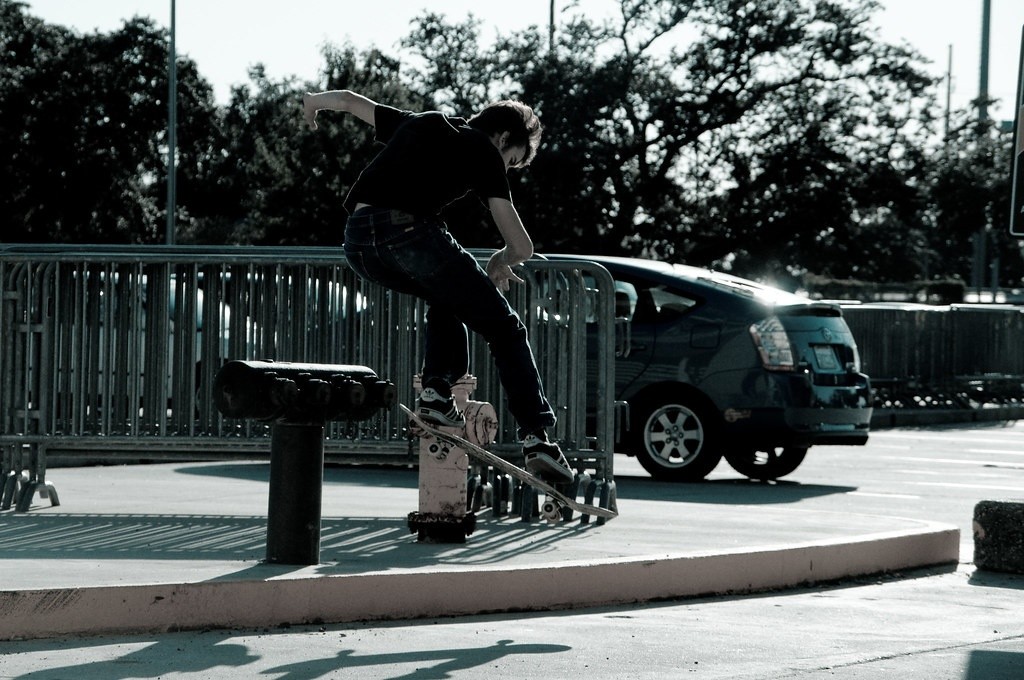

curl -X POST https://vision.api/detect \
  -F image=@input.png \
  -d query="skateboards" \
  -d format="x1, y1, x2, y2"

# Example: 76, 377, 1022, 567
399, 403, 617, 524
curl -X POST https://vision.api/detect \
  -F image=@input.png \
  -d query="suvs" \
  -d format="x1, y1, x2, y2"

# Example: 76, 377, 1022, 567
279, 252, 872, 484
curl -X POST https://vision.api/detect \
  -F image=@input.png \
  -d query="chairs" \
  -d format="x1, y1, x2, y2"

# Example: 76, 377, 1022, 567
616, 290, 630, 319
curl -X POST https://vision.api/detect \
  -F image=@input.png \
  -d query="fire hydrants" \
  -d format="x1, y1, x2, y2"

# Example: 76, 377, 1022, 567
403, 356, 499, 544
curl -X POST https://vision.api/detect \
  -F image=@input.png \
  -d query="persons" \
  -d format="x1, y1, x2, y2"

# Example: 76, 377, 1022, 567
302, 90, 578, 488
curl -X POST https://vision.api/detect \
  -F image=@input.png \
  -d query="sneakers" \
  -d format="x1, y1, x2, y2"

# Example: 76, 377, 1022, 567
521, 436, 574, 484
417, 388, 466, 428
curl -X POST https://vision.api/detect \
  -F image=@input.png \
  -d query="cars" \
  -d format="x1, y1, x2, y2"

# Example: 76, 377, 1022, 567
162, 269, 375, 340
0, 261, 282, 437
